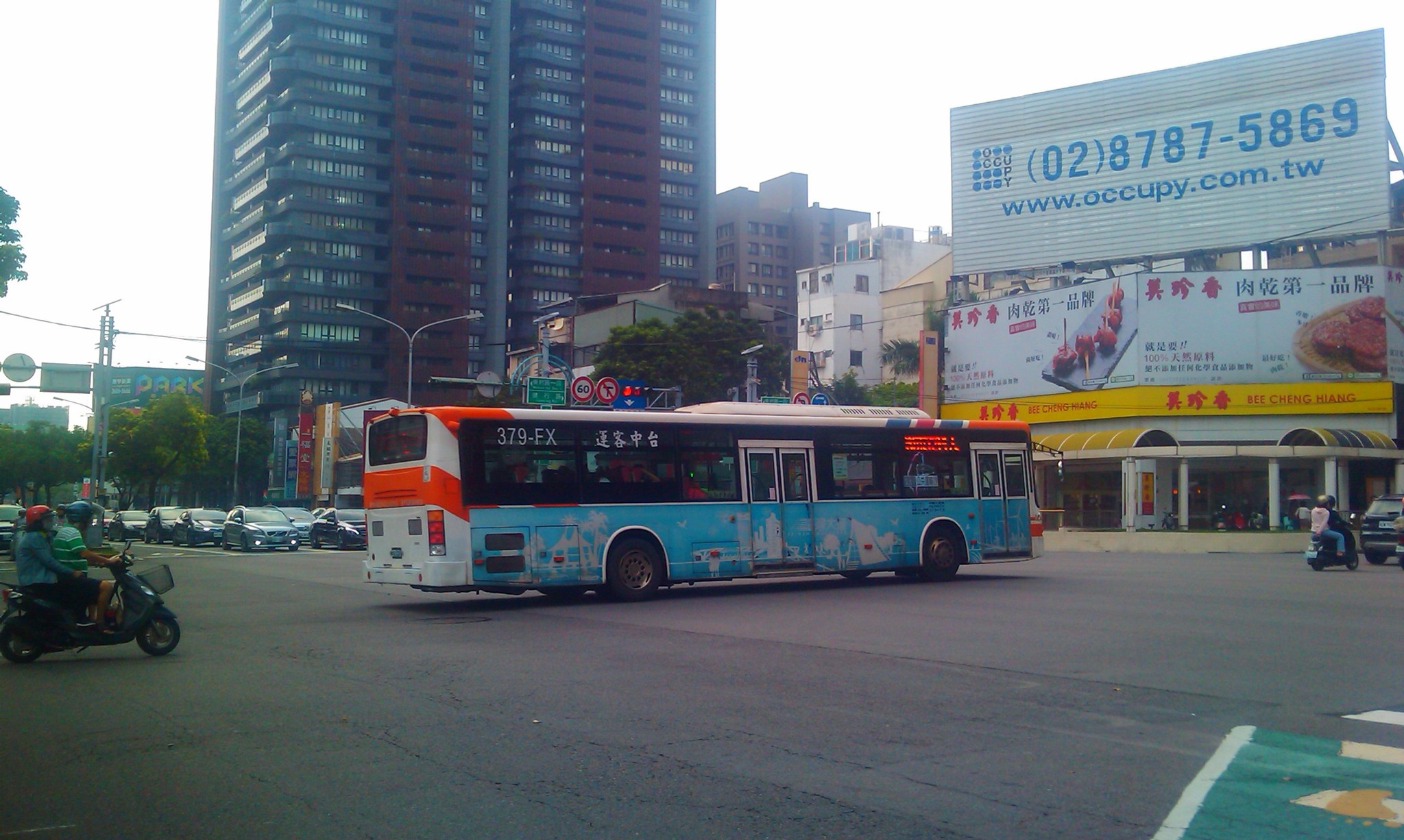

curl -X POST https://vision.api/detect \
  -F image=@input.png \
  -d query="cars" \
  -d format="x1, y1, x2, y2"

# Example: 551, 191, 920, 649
92, 509, 118, 536
222, 505, 302, 553
108, 510, 150, 542
172, 506, 228, 548
308, 507, 368, 551
0, 505, 24, 551
278, 505, 329, 542
144, 506, 190, 545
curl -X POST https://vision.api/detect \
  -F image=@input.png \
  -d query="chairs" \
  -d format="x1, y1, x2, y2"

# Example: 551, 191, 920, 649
471, 463, 903, 502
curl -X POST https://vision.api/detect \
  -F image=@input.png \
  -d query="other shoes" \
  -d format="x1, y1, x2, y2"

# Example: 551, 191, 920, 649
98, 627, 115, 636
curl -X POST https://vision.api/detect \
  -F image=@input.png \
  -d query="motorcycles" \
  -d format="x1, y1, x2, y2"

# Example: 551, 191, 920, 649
0, 538, 180, 664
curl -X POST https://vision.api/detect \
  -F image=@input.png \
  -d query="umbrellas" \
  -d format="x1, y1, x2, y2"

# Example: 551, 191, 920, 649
1287, 493, 1310, 500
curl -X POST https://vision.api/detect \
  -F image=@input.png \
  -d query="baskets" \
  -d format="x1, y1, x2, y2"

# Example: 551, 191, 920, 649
136, 564, 175, 594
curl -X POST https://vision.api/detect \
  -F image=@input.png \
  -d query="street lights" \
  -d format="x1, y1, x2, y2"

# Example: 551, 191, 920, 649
336, 302, 484, 408
186, 356, 300, 506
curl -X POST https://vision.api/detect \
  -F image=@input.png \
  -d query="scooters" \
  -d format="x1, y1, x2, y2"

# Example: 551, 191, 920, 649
10, 522, 27, 550
1212, 504, 1228, 532
1303, 511, 1359, 571
1161, 511, 1179, 529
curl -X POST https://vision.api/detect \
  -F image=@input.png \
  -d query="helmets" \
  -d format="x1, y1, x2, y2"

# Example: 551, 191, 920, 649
57, 504, 66, 511
1327, 495, 1335, 507
26, 505, 58, 531
66, 501, 93, 523
18, 508, 27, 516
1315, 495, 1329, 507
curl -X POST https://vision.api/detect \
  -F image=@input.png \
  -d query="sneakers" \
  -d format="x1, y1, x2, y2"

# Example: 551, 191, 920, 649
76, 616, 98, 626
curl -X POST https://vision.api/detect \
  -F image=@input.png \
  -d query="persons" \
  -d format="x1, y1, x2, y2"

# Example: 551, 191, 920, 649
8, 508, 27, 561
15, 505, 97, 627
1311, 494, 1345, 556
52, 503, 68, 528
1295, 502, 1311, 533
51, 500, 124, 635
1327, 495, 1353, 533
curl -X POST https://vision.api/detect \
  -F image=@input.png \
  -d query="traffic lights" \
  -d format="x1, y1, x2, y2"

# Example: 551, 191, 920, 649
620, 383, 654, 398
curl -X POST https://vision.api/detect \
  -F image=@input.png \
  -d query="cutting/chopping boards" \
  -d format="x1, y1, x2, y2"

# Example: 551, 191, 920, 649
1042, 289, 1142, 392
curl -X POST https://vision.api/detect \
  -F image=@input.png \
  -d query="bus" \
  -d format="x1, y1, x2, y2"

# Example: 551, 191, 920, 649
362, 401, 1066, 603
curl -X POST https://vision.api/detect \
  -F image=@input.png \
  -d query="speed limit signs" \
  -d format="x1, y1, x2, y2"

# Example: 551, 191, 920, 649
571, 375, 596, 403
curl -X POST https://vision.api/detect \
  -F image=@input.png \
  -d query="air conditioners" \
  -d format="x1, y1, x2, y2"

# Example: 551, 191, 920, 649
808, 325, 820, 335
822, 275, 831, 281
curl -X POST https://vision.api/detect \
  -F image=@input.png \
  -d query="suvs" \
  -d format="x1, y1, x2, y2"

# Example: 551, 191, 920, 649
1359, 492, 1404, 565
